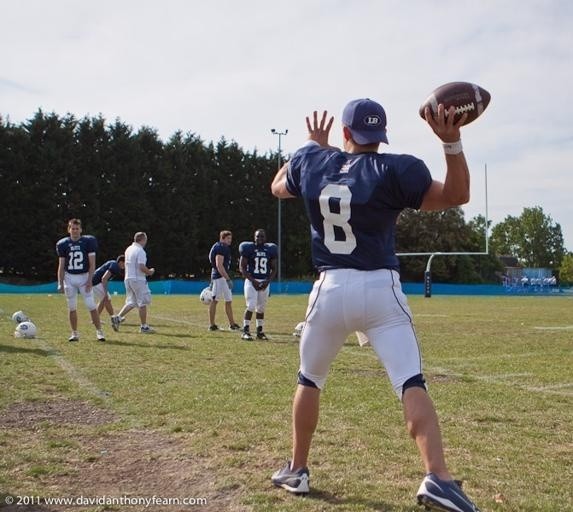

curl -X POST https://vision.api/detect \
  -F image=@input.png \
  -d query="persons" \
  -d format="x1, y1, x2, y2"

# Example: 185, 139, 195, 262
91, 254, 126, 328
207, 229, 242, 331
501, 272, 558, 293
54, 219, 106, 343
111, 232, 158, 334
238, 228, 279, 342
270, 96, 487, 512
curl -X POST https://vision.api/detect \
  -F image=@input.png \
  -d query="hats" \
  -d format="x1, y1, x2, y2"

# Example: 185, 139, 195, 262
341, 96, 389, 146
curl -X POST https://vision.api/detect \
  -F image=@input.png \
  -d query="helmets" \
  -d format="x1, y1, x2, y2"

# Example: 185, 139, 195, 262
199, 287, 214, 306
292, 321, 305, 338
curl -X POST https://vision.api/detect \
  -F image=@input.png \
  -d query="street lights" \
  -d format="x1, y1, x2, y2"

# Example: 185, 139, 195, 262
268, 127, 288, 292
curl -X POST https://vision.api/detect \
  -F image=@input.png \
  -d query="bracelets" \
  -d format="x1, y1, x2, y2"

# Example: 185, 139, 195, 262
439, 140, 463, 155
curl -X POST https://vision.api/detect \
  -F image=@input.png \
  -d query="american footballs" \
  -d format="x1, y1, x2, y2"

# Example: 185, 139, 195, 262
419, 82, 490, 126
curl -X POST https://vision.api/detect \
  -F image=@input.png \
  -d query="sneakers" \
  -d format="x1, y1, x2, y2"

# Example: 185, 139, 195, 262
271, 465, 309, 494
111, 315, 122, 333
140, 325, 156, 333
229, 323, 244, 331
208, 324, 224, 331
416, 474, 483, 511
241, 329, 267, 342
69, 330, 79, 341
96, 329, 105, 340
